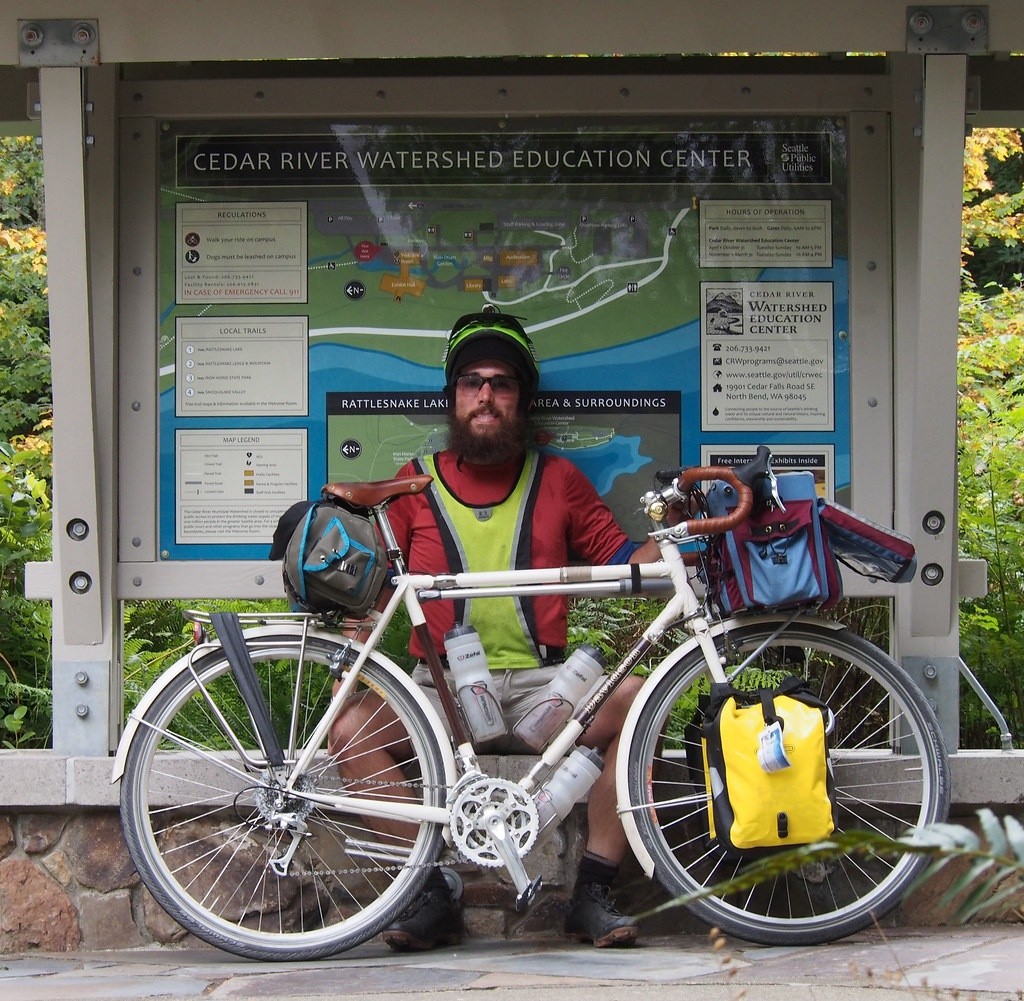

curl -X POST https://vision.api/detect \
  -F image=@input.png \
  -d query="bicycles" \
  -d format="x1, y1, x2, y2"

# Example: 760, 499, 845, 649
111, 445, 951, 962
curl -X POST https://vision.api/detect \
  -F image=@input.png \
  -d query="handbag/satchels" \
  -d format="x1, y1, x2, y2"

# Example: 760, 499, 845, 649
702, 471, 917, 616
688, 680, 838, 851
270, 501, 387, 620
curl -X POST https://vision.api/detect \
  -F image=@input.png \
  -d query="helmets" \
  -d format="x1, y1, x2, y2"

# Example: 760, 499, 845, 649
442, 313, 539, 390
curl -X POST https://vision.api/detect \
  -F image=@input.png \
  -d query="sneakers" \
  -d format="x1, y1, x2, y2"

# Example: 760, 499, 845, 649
382, 888, 464, 950
564, 882, 642, 948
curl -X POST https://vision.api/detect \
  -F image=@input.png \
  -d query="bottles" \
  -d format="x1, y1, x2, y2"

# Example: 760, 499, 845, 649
443, 621, 506, 743
514, 643, 608, 752
516, 745, 606, 852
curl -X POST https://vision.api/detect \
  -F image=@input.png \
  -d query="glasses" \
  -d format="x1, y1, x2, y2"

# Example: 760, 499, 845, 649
456, 374, 521, 393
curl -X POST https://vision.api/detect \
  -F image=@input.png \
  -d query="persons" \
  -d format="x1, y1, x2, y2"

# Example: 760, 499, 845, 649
327, 312, 707, 947
